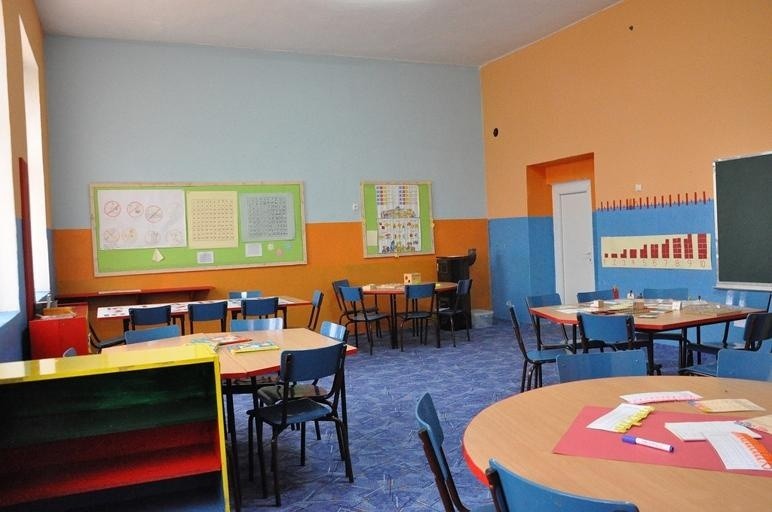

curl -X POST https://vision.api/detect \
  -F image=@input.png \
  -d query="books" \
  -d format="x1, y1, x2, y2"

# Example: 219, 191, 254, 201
665, 421, 762, 441
363, 283, 404, 290
688, 399, 765, 413
193, 333, 280, 354
558, 298, 742, 319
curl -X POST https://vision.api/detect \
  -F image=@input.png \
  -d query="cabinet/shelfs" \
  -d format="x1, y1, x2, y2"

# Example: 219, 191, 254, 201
0, 342, 231, 512
29, 302, 90, 360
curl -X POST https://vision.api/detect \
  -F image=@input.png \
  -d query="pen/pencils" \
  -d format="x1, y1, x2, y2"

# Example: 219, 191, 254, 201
622, 433, 674, 453
628, 290, 633, 297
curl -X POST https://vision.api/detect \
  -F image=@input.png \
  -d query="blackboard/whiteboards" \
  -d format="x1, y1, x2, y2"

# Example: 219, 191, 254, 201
712, 151, 772, 291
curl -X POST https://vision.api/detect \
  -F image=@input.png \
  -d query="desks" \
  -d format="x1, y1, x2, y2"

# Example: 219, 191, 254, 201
100, 327, 360, 479
463, 376, 770, 511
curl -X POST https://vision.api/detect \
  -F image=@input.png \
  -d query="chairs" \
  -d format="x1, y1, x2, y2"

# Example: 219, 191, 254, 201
413, 392, 464, 510
257, 320, 351, 473
331, 276, 495, 354
505, 276, 772, 392
61, 278, 324, 352
485, 458, 640, 512
248, 341, 355, 506
